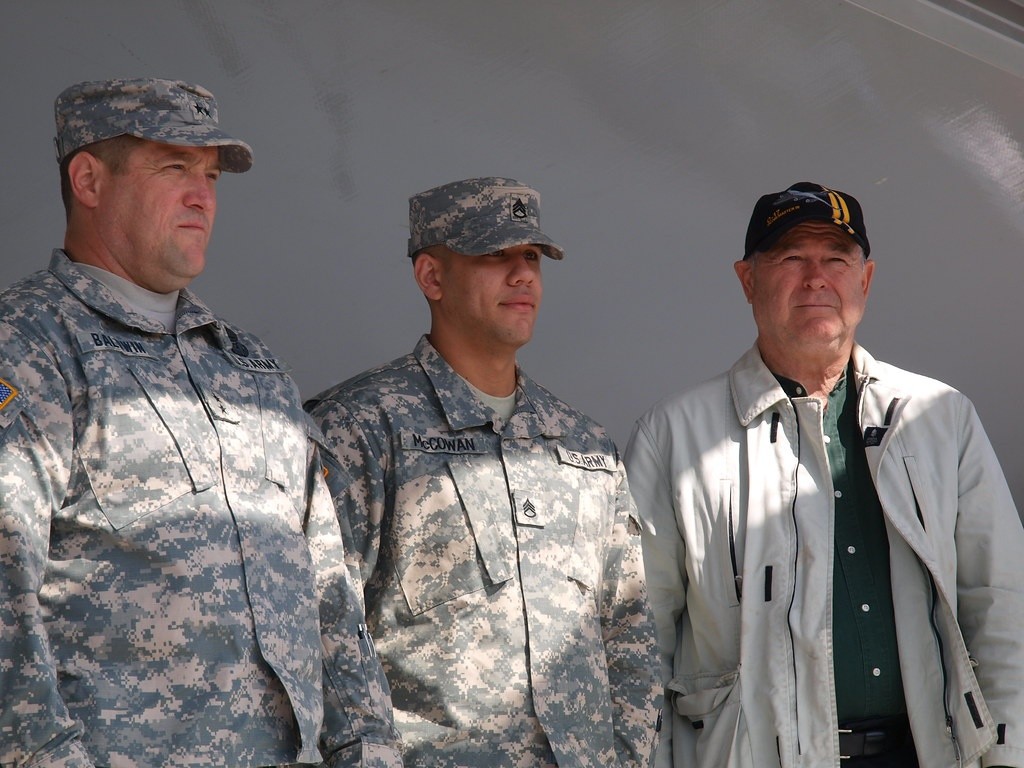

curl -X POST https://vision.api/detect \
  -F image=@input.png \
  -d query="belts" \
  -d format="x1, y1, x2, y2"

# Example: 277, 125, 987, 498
837, 723, 914, 760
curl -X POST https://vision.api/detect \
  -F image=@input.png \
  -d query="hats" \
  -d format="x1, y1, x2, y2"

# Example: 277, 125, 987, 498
53, 77, 253, 173
743, 182, 870, 260
406, 177, 565, 259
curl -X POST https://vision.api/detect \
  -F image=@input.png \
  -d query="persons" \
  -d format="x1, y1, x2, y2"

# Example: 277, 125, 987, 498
624, 181, 1024, 768
0, 79, 405, 768
302, 176, 665, 768
